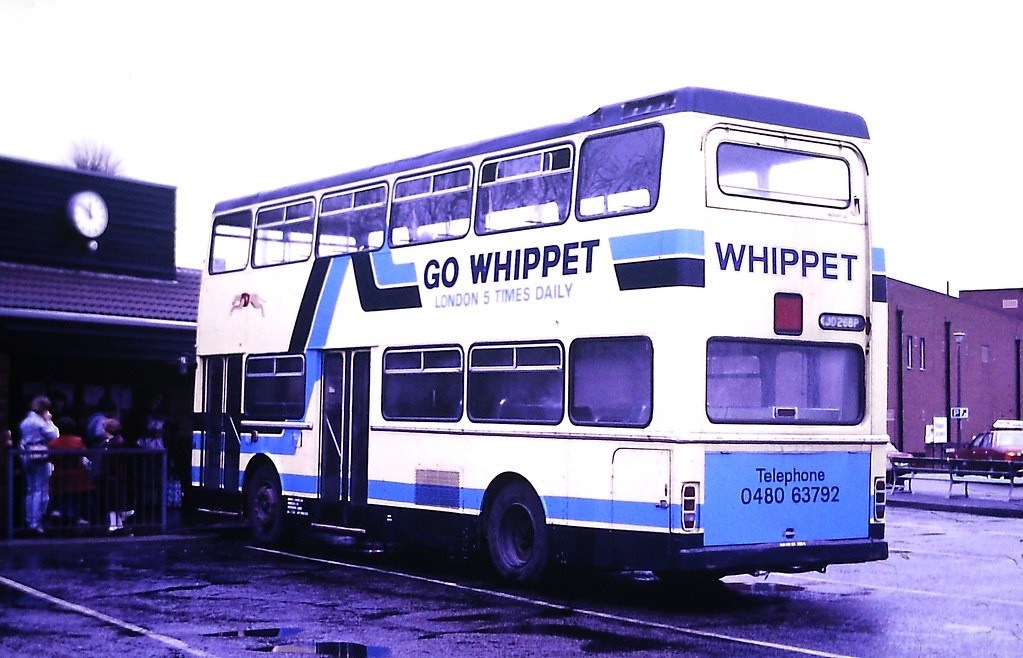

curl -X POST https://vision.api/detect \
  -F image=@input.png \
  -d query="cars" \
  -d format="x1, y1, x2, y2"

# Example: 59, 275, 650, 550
886, 442, 914, 484
947, 428, 1023, 479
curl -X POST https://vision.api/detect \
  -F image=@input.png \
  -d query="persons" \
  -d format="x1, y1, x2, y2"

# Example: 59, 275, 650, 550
20, 395, 63, 530
47, 418, 92, 532
92, 419, 132, 536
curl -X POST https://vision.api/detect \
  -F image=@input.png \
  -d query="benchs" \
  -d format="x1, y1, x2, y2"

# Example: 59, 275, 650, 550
424, 398, 652, 427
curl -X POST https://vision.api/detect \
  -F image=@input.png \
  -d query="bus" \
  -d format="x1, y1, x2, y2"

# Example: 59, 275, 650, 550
189, 86, 891, 585
